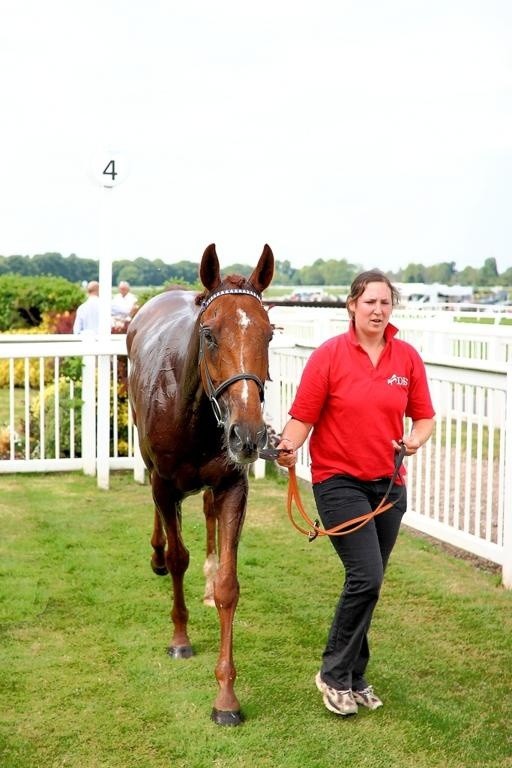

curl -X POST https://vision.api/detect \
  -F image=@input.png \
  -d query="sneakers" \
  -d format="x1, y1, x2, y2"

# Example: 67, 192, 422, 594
315, 670, 384, 716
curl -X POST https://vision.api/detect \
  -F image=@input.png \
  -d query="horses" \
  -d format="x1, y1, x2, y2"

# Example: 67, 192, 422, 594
122, 241, 275, 728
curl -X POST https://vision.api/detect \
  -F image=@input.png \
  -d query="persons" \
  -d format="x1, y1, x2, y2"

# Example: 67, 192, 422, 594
72, 280, 137, 334
276, 271, 436, 716
